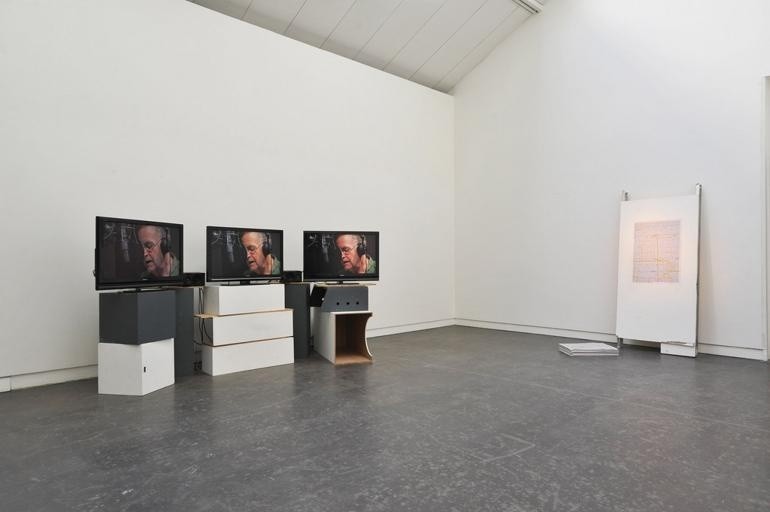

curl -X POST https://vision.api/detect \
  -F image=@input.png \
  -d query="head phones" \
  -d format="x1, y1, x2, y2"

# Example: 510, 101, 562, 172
161, 227, 170, 254
262, 232, 273, 256
357, 233, 367, 256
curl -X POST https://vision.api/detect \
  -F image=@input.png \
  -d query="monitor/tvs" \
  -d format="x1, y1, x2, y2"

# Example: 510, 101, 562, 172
206, 225, 283, 286
303, 230, 379, 285
96, 217, 183, 293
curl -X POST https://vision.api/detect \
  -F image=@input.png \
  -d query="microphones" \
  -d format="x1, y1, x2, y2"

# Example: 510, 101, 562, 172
226, 243, 234, 262
323, 246, 329, 264
121, 239, 130, 263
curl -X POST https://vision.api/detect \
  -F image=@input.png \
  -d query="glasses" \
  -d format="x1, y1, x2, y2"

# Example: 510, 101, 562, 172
143, 238, 163, 256
248, 243, 265, 256
341, 244, 358, 256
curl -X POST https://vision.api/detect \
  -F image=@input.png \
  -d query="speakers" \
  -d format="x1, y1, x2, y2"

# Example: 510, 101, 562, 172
280, 271, 301, 282
310, 285, 327, 307
182, 273, 204, 286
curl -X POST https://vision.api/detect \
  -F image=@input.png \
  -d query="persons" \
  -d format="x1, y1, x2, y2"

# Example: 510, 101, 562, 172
136, 223, 181, 281
336, 233, 377, 277
239, 230, 281, 277
